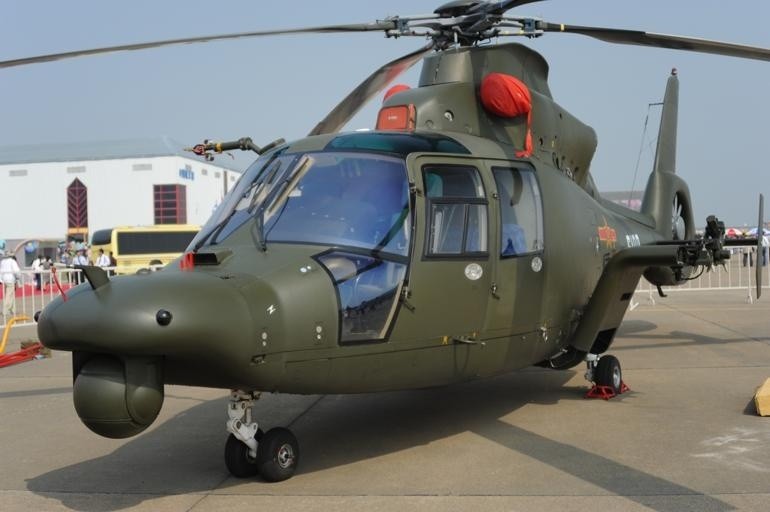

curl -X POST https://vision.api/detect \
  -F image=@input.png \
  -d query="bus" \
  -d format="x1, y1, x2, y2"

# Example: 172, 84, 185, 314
88, 222, 203, 276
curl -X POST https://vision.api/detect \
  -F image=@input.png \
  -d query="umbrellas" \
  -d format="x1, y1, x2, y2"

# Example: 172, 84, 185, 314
723, 228, 743, 236
748, 227, 770, 234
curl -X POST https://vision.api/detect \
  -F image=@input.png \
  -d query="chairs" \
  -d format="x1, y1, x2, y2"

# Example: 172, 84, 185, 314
352, 173, 444, 301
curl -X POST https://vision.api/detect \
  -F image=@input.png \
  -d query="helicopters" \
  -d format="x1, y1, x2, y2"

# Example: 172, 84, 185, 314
0, 0, 770, 484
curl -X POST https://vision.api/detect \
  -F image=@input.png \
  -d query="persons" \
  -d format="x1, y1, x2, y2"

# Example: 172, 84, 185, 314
741, 246, 754, 266
761, 232, 770, 264
0, 249, 21, 315
31, 247, 117, 292
